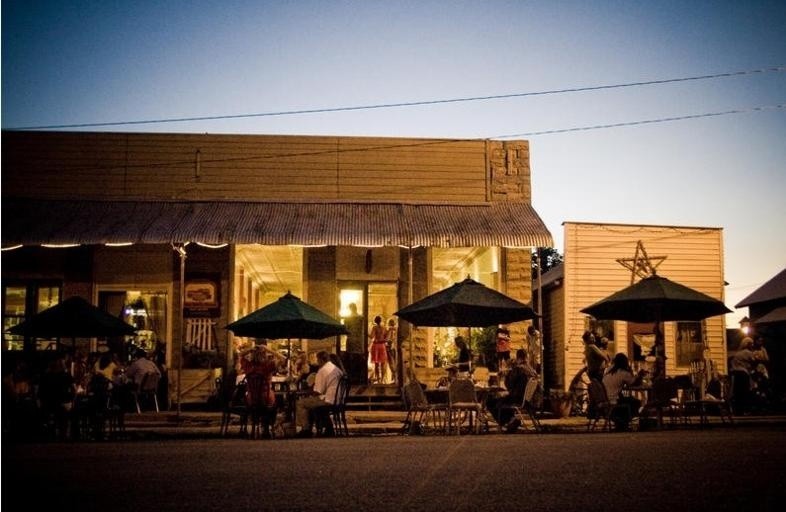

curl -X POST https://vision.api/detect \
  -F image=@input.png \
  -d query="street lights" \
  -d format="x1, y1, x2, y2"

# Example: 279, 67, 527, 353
171, 242, 189, 420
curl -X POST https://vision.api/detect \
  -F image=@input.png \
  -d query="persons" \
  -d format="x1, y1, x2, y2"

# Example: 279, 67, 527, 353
346, 303, 398, 382
231, 339, 346, 435
730, 335, 769, 417
455, 324, 541, 427
10, 348, 164, 435
583, 328, 640, 428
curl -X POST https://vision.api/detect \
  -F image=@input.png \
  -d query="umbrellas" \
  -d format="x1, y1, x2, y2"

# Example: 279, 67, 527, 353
753, 305, 786, 324
734, 269, 786, 308
10, 295, 137, 362
393, 277, 536, 371
580, 269, 734, 355
225, 290, 346, 361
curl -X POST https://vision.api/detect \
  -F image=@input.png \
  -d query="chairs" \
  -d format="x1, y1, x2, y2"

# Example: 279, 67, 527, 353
402, 372, 544, 435
9, 378, 139, 443
588, 366, 737, 433
215, 373, 352, 438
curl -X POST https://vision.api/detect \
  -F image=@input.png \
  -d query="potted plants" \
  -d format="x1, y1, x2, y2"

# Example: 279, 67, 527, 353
550, 389, 575, 417
